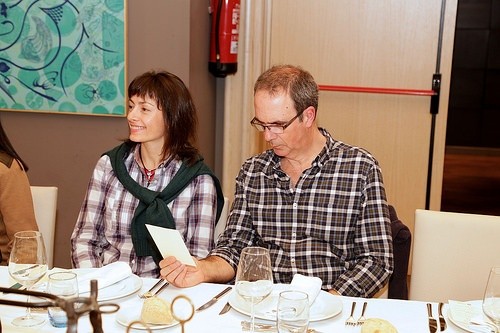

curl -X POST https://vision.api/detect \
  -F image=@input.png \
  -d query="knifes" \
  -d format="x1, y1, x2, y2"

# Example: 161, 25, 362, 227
218, 303, 231, 315
426, 302, 447, 333
195, 287, 232, 311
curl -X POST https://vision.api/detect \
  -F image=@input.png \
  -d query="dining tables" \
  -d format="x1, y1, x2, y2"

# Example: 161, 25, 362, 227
0, 264, 471, 333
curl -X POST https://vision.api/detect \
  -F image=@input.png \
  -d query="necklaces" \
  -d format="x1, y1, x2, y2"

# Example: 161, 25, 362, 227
137, 142, 164, 177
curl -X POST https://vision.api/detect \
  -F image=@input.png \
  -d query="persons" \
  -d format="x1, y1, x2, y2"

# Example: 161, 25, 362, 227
0, 120, 38, 266
70, 70, 224, 280
159, 65, 394, 298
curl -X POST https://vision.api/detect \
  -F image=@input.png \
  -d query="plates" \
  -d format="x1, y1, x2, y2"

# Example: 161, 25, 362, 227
115, 302, 180, 330
227, 284, 343, 322
66, 268, 143, 302
447, 299, 495, 333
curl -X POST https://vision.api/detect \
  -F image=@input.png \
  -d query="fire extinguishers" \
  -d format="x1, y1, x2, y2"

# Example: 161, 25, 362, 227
207, 0, 240, 78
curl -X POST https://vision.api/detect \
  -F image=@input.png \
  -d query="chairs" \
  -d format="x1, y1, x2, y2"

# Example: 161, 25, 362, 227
409, 210, 499, 302
30, 186, 58, 269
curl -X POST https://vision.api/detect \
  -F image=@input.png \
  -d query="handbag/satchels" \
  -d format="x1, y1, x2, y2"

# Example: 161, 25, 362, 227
388, 203, 412, 299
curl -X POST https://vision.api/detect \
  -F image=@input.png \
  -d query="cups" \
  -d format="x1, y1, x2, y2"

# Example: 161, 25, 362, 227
276, 291, 310, 333
48, 272, 79, 329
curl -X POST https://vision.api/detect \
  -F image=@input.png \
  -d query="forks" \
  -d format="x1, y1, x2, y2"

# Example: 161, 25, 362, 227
344, 301, 367, 326
138, 278, 169, 299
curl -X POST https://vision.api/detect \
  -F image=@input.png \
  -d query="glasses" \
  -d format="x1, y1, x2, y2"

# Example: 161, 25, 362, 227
250, 110, 301, 133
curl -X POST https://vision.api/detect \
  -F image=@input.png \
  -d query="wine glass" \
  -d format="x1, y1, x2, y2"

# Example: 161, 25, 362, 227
10, 230, 48, 328
234, 246, 273, 333
482, 266, 500, 333
